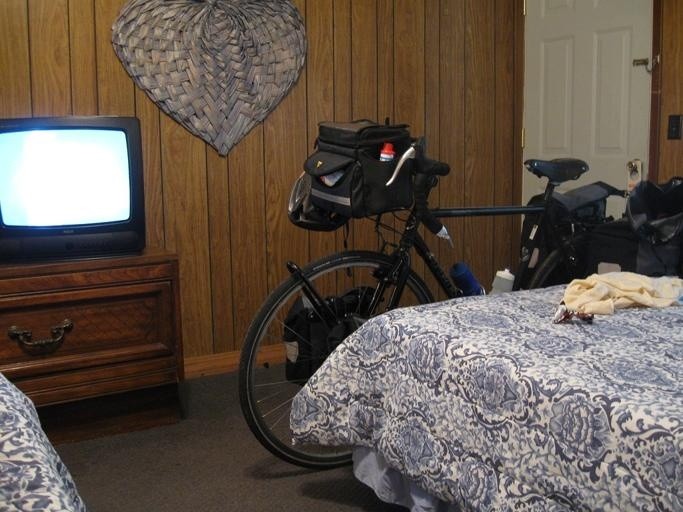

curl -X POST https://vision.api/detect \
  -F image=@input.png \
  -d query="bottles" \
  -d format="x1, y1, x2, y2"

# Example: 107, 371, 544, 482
488, 268, 517, 296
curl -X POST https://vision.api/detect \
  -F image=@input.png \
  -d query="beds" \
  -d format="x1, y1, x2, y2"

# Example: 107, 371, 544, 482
372, 279, 683, 510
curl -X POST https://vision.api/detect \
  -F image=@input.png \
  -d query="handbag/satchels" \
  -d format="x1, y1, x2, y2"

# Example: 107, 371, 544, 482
284, 286, 384, 386
518, 181, 616, 251
309, 117, 413, 218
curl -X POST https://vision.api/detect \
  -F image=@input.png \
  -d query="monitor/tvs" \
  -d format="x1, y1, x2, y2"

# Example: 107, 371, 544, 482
0, 117, 147, 262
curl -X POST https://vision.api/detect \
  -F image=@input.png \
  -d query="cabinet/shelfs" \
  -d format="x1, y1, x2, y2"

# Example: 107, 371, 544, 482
1, 246, 188, 415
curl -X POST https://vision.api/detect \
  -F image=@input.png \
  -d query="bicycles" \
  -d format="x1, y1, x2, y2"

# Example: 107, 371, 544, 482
237, 155, 637, 468
